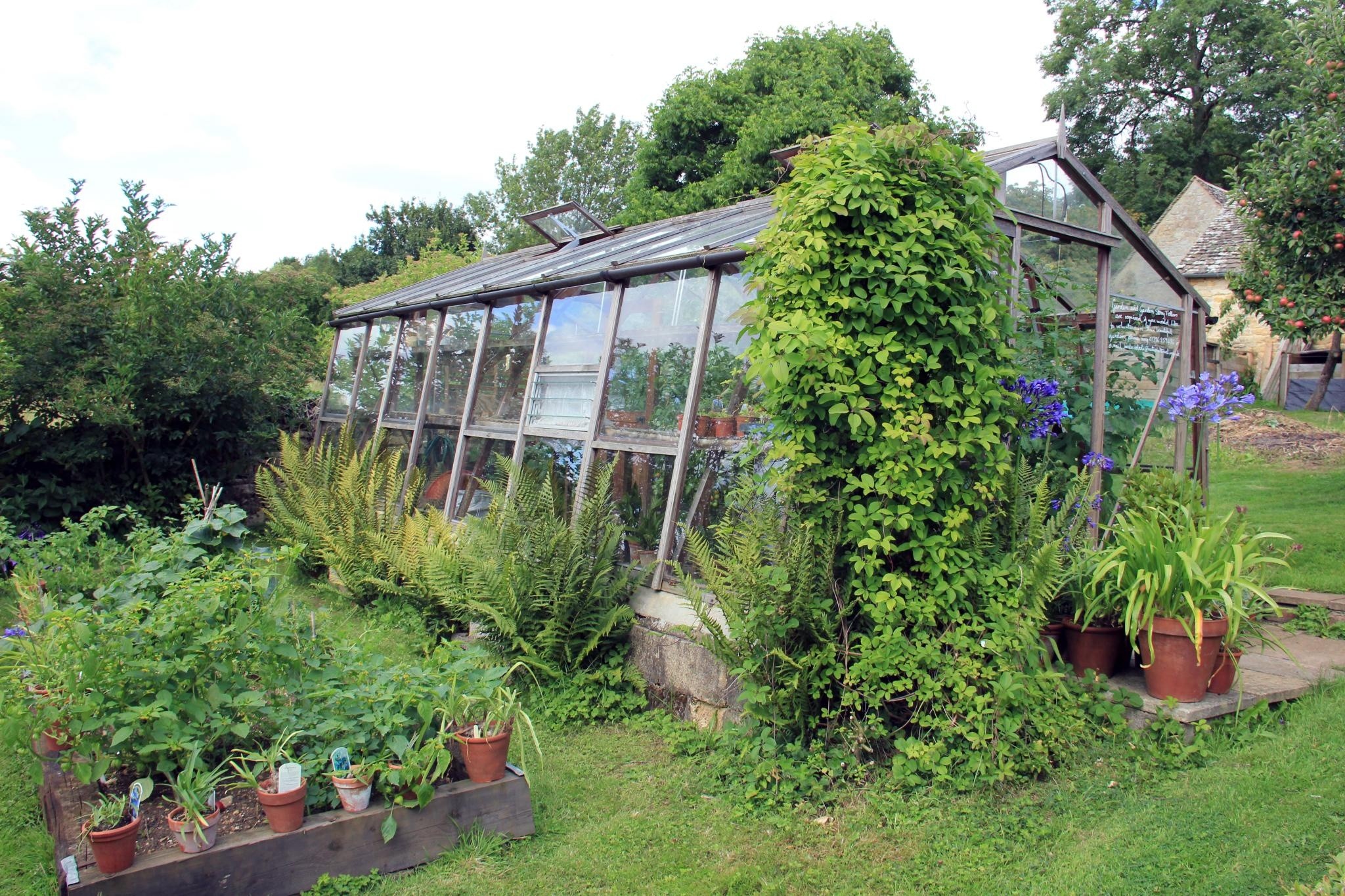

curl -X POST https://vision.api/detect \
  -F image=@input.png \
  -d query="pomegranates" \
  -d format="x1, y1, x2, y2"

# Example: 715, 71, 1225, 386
1238, 57, 1345, 332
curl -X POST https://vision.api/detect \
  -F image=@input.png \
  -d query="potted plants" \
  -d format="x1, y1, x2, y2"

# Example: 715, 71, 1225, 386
1061, 542, 1136, 673
1204, 584, 1310, 697
606, 335, 769, 434
1, 476, 548, 877
1096, 488, 1294, 694
